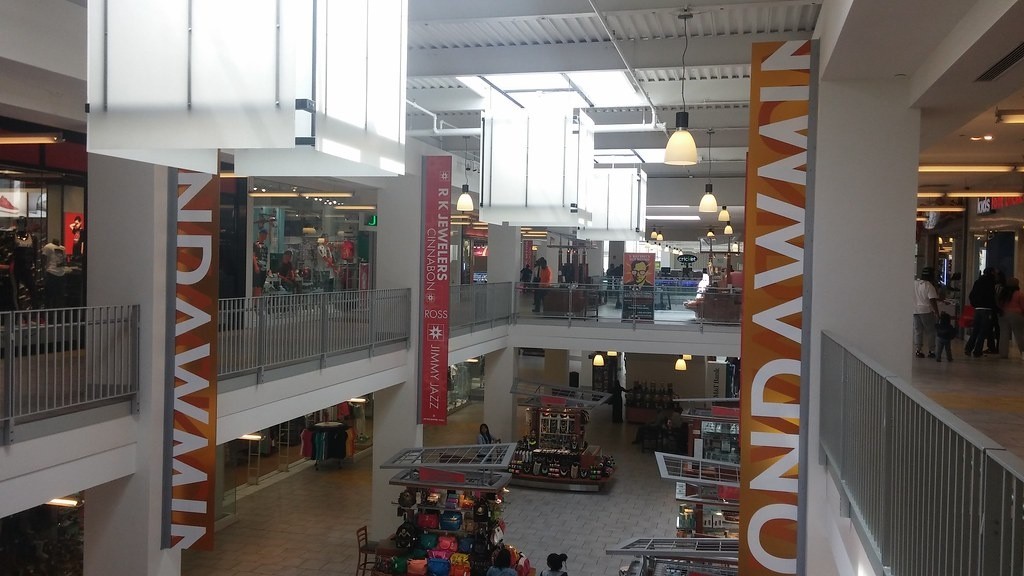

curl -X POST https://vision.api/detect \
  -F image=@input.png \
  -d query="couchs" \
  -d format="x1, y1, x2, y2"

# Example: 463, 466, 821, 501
542, 286, 588, 318
702, 288, 743, 322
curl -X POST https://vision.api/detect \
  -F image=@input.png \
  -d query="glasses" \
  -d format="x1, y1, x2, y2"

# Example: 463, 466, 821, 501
632, 270, 646, 275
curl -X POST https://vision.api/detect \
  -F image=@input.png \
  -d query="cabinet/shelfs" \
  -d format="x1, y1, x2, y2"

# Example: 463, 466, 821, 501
337, 256, 366, 311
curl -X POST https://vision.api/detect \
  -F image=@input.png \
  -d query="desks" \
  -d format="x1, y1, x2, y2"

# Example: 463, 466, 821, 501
626, 405, 659, 424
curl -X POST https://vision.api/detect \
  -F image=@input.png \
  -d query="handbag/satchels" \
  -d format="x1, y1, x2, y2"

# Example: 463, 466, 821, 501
533, 277, 540, 283
958, 304, 975, 328
392, 487, 533, 576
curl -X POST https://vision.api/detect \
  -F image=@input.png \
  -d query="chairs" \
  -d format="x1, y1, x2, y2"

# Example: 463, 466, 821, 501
356, 526, 378, 576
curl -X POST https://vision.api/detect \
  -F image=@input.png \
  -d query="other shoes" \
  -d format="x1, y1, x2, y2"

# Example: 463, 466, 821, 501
915, 347, 1009, 362
532, 310, 540, 312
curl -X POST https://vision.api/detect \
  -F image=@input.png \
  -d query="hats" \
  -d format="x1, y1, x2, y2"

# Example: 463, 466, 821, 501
1006, 278, 1019, 286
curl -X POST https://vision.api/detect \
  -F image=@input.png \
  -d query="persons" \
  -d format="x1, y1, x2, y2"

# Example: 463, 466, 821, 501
476, 423, 501, 461
485, 549, 519, 576
656, 264, 736, 294
632, 405, 665, 445
912, 266, 942, 359
605, 262, 623, 295
69, 215, 85, 257
662, 407, 681, 439
520, 254, 551, 312
254, 226, 352, 305
936, 257, 1024, 363
539, 553, 569, 576
0, 215, 69, 324
558, 263, 586, 287
609, 379, 632, 424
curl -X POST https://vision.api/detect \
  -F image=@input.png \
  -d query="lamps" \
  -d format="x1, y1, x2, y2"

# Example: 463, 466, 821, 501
683, 355, 693, 360
645, 241, 651, 248
607, 351, 616, 356
731, 243, 740, 256
718, 206, 730, 222
672, 247, 677, 253
592, 351, 605, 367
651, 228, 658, 239
658, 243, 662, 251
675, 249, 679, 255
723, 222, 733, 235
698, 130, 719, 213
663, 10, 698, 166
669, 246, 673, 253
664, 245, 669, 252
707, 228, 715, 237
679, 249, 683, 255
675, 354, 688, 371
654, 242, 658, 251
657, 230, 663, 241
456, 138, 475, 212
